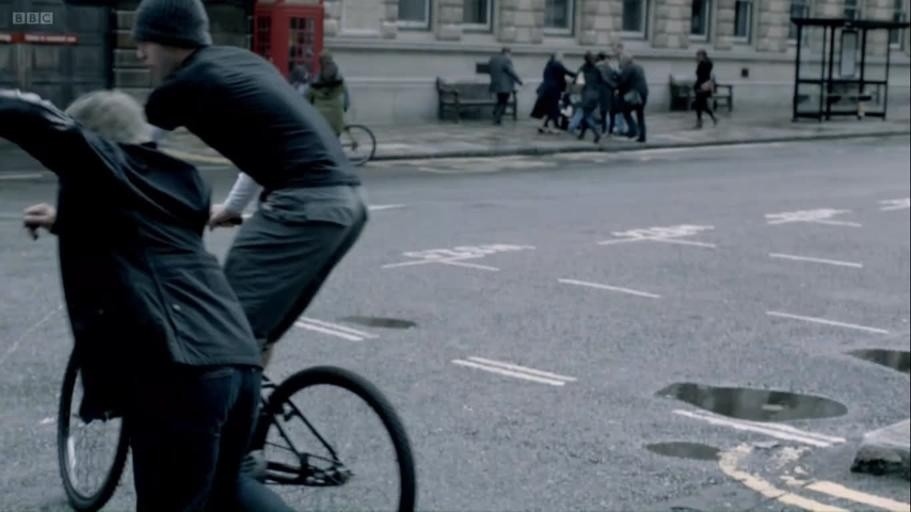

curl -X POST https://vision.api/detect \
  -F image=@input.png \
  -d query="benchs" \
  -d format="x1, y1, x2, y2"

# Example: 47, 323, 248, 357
669, 74, 733, 112
435, 76, 518, 124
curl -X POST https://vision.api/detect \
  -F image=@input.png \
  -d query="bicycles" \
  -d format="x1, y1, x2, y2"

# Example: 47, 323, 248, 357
336, 122, 376, 167
24, 215, 415, 512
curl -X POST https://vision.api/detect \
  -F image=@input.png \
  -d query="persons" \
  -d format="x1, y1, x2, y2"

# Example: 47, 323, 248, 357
489, 45, 522, 124
1, 88, 294, 512
290, 48, 349, 137
132, 2, 369, 479
692, 50, 717, 128
531, 44, 648, 142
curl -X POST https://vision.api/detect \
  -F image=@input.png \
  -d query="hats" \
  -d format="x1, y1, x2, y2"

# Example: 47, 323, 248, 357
132, 0, 214, 48
